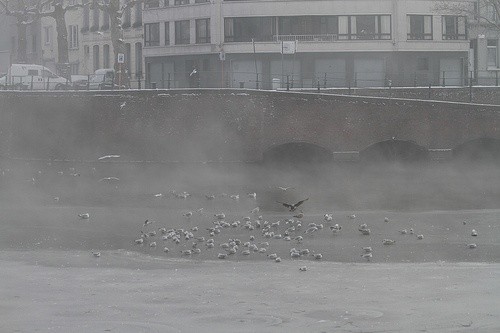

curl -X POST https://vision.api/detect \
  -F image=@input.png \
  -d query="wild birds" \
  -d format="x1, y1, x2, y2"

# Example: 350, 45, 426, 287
1, 66, 479, 272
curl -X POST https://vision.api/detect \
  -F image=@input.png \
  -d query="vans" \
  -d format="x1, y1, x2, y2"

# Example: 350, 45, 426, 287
0, 64, 113, 91
